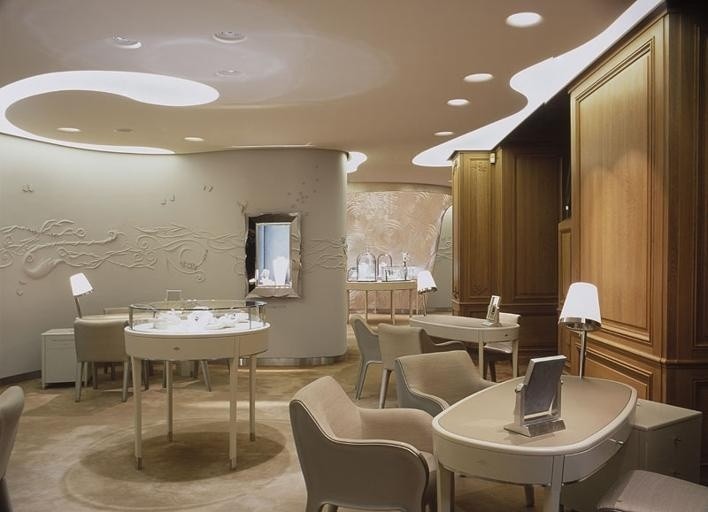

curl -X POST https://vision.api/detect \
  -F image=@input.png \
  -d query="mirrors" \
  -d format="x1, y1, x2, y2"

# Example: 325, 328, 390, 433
243, 210, 303, 300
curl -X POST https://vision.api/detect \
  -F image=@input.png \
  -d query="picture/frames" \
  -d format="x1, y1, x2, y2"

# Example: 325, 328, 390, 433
485, 295, 502, 322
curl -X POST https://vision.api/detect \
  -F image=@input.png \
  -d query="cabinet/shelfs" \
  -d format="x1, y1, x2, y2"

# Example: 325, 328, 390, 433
41, 328, 92, 389
559, 397, 703, 511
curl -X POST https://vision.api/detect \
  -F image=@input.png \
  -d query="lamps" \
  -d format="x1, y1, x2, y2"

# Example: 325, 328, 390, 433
557, 281, 602, 376
416, 270, 438, 317
70, 272, 94, 319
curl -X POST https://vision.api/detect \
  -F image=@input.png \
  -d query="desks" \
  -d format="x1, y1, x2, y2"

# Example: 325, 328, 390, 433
431, 374, 638, 512
124, 298, 272, 470
346, 280, 417, 325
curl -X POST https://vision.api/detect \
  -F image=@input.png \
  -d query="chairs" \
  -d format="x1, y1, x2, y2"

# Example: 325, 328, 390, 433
73, 307, 154, 403
